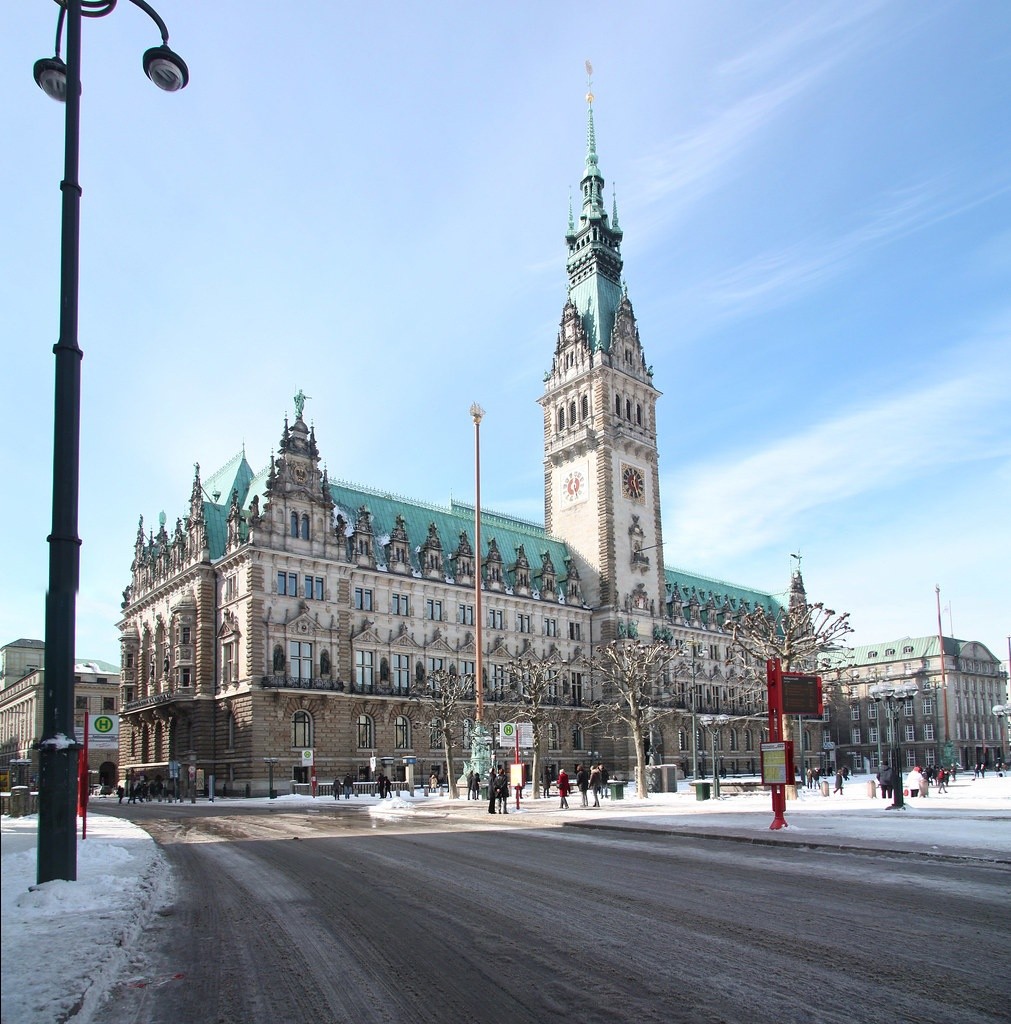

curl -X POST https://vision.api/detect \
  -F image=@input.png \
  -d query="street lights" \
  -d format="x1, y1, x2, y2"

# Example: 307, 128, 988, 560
697, 712, 730, 796
262, 756, 278, 799
866, 679, 919, 810
456, 402, 495, 794
33, 1, 189, 886
1006, 631, 1011, 698
678, 635, 709, 790
416, 758, 427, 789
934, 583, 964, 774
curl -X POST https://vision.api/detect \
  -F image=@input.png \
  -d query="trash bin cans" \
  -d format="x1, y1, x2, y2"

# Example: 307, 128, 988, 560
696, 783, 710, 800
482, 786, 488, 800
610, 784, 623, 800
272, 790, 277, 798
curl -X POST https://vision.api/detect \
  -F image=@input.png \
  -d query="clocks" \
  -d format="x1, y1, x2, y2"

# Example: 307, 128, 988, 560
559, 460, 590, 512
619, 458, 647, 508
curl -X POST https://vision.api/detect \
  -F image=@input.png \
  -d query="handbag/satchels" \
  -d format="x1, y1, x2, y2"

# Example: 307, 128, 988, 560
556, 782, 561, 788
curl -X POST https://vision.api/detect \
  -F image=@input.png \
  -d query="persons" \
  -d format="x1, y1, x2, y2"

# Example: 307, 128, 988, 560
588, 765, 602, 807
488, 767, 498, 813
556, 768, 571, 809
833, 769, 844, 795
542, 766, 552, 799
599, 765, 609, 799
806, 765, 850, 789
344, 773, 354, 799
576, 765, 588, 807
116, 779, 163, 804
471, 773, 480, 800
467, 770, 473, 800
905, 760, 1008, 798
493, 769, 509, 814
377, 772, 438, 798
332, 776, 343, 800
876, 761, 894, 798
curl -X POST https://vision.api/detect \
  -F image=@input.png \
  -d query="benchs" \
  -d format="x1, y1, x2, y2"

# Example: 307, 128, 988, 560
688, 781, 803, 801
539, 782, 627, 801
